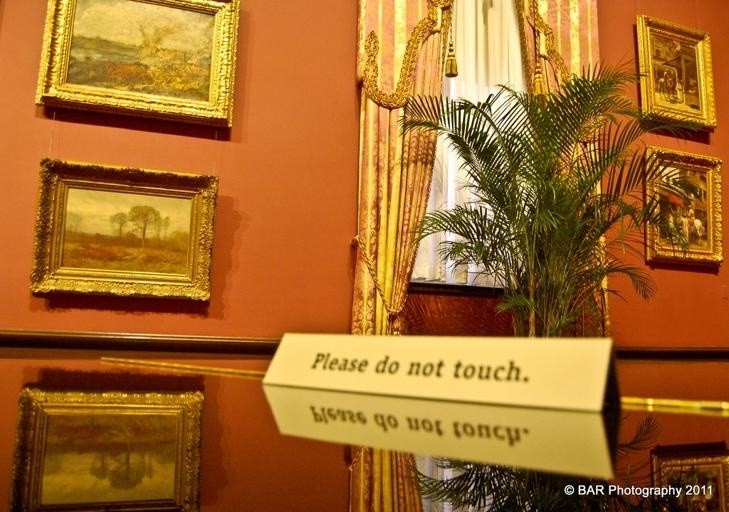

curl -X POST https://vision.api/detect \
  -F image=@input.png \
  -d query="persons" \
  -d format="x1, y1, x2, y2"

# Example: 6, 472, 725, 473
660, 68, 697, 105
667, 204, 703, 243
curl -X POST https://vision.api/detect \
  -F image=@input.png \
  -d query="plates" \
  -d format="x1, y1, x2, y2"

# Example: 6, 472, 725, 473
29, 158, 219, 302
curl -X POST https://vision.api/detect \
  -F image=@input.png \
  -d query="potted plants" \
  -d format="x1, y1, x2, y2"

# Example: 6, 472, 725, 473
636, 15, 717, 133
12, 388, 204, 512
646, 146, 724, 267
650, 453, 729, 512
35, 0, 243, 128
